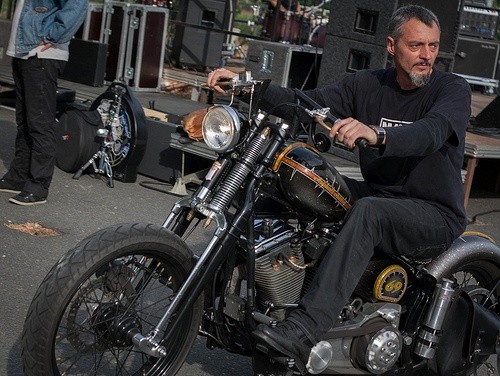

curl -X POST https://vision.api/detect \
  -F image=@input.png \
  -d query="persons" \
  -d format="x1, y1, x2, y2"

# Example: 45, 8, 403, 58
254, 0, 301, 42
0, 0, 89, 206
208, 4, 471, 374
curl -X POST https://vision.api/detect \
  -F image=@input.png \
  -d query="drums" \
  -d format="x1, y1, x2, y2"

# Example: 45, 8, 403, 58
266, 9, 311, 43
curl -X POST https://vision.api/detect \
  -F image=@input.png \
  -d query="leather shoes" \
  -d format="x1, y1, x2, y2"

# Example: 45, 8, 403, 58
251, 320, 312, 374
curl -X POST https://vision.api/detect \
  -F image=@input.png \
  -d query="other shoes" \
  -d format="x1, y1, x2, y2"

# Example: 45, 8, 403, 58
0, 178, 22, 193
9, 191, 47, 205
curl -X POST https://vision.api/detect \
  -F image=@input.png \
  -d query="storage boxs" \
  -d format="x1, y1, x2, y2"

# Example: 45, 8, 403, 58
76, 0, 169, 92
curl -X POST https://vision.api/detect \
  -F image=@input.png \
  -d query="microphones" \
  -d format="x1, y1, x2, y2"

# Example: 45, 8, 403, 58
456, 52, 466, 58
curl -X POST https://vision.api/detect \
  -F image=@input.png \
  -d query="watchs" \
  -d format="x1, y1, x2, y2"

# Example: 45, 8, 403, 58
366, 124, 387, 148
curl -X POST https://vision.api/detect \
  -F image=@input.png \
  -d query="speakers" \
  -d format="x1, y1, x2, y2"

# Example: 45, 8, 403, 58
316, 0, 500, 89
173, 0, 229, 67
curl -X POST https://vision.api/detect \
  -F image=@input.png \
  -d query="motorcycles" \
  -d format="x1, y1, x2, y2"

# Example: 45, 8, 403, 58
19, 71, 500, 376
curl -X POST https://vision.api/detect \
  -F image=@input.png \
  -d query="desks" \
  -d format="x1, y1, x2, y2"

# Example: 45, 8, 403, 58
462, 133, 500, 211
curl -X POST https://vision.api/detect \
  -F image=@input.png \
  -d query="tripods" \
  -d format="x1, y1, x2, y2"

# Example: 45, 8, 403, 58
72, 94, 122, 188
